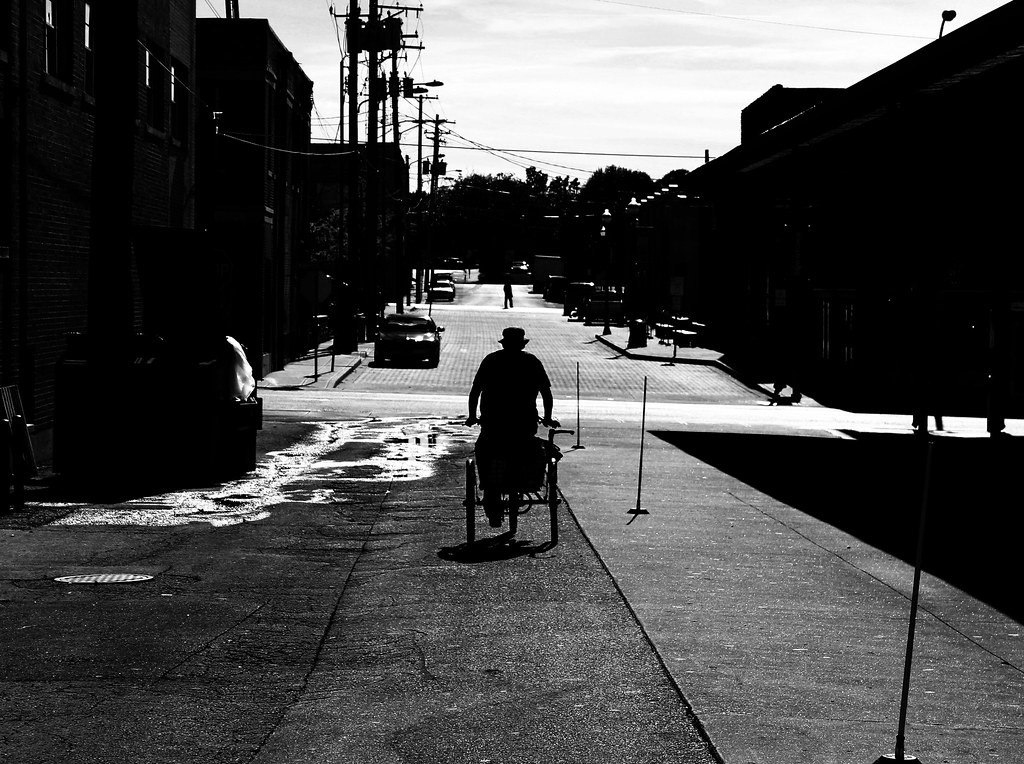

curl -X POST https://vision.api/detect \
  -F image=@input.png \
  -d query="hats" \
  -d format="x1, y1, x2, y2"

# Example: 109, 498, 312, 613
497, 327, 531, 347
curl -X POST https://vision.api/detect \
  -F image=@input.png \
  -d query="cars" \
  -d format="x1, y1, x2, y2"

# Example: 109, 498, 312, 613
375, 312, 440, 367
562, 281, 630, 327
427, 275, 454, 304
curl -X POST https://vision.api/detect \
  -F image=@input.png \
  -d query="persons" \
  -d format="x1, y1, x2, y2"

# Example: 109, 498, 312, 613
504, 280, 513, 309
464, 327, 561, 528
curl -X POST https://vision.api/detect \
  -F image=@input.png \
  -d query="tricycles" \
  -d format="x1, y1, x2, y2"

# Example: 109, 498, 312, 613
459, 414, 576, 546
